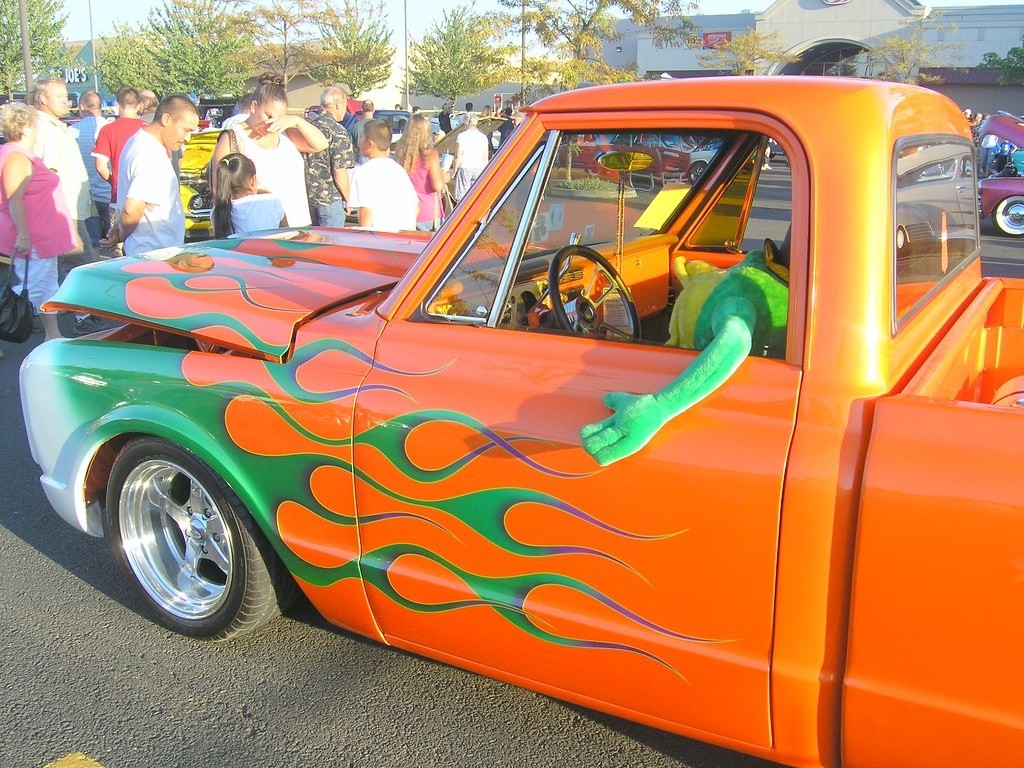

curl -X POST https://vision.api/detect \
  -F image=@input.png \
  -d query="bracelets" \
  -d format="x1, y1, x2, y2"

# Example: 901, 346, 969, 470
17, 235, 31, 240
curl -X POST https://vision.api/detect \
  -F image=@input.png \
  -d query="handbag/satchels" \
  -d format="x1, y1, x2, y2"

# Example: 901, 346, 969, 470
0, 248, 34, 343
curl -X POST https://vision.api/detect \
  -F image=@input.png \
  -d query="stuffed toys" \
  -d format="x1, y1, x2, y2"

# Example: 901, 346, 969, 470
578, 223, 791, 467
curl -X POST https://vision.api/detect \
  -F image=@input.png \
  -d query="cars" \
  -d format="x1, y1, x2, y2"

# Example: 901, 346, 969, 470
178, 131, 223, 242
433, 116, 522, 204
685, 132, 725, 185
896, 144, 977, 259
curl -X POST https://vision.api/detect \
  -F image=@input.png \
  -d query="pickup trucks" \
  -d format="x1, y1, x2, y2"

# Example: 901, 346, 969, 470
18, 75, 1024, 768
355, 108, 411, 147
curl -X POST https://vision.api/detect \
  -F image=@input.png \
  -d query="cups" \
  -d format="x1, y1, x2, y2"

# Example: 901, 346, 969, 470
440, 154, 454, 172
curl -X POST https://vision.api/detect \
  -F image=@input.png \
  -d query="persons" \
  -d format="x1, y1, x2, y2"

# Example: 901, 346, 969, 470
210, 72, 451, 234
451, 102, 534, 211
0, 101, 79, 344
99, 95, 201, 256
210, 153, 289, 239
20, 76, 183, 338
961, 108, 1018, 180
